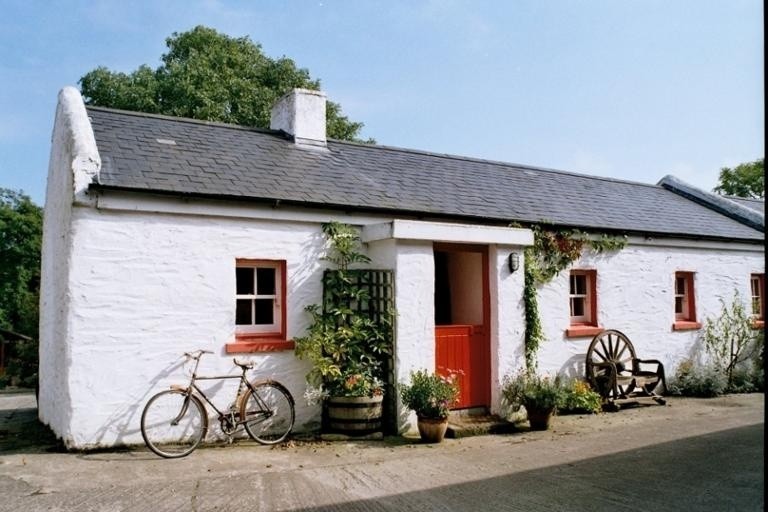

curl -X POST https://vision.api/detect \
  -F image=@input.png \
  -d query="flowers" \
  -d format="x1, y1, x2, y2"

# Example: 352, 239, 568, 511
293, 218, 395, 407
568, 380, 600, 416
398, 366, 463, 422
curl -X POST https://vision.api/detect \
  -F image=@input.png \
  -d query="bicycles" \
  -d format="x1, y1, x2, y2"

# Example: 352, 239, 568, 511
138, 348, 297, 461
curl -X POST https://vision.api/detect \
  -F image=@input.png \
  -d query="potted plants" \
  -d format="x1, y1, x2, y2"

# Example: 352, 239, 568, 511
501, 367, 565, 439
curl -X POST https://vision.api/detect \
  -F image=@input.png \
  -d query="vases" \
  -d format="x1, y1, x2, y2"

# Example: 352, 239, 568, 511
418, 416, 451, 443
329, 396, 386, 436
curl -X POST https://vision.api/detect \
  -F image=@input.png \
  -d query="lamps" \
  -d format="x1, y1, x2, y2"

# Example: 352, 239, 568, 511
510, 252, 520, 272
